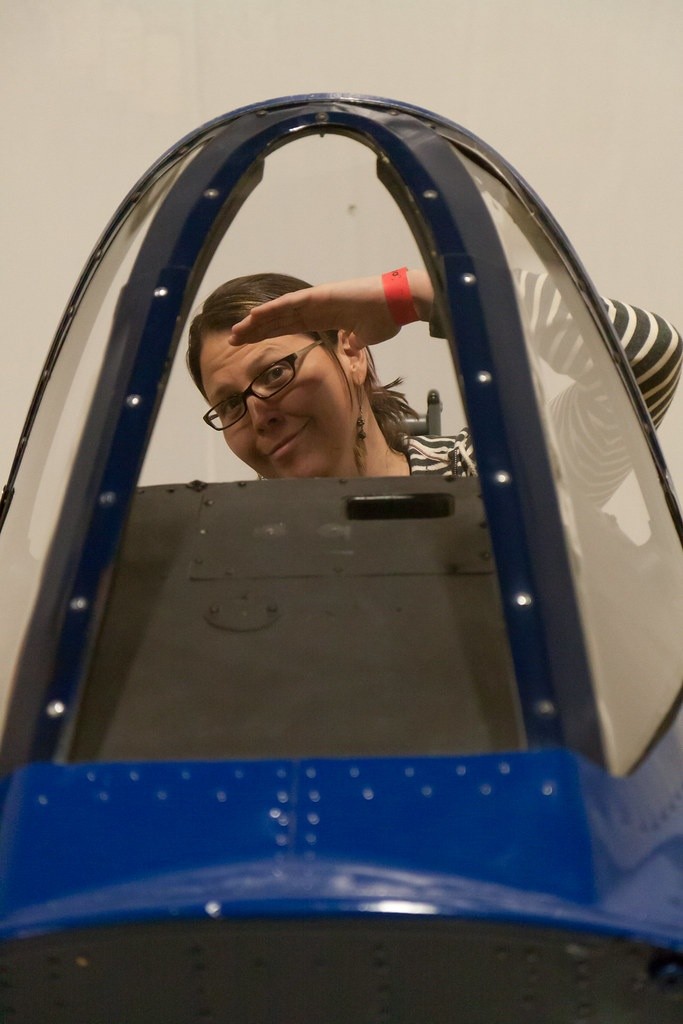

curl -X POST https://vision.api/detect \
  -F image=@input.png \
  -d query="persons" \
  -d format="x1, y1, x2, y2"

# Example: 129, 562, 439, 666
186, 268, 683, 510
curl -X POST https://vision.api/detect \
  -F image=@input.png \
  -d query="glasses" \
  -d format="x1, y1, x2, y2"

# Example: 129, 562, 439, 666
202, 340, 323, 431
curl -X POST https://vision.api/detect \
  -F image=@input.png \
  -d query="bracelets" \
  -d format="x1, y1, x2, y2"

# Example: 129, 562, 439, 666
381, 268, 421, 328
